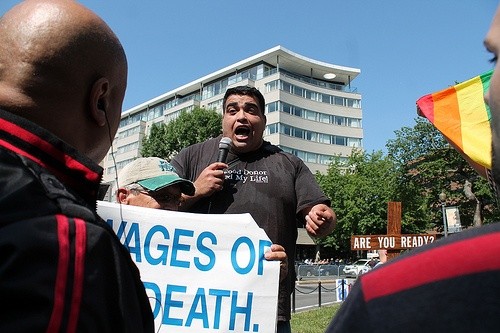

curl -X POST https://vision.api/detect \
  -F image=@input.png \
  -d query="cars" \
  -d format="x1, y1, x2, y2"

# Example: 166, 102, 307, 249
294, 260, 344, 277
343, 258, 381, 278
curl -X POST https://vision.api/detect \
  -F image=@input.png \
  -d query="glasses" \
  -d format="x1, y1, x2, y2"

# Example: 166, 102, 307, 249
134, 189, 182, 203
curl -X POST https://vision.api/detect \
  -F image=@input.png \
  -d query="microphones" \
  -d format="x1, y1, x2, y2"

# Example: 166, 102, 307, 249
217, 137, 232, 170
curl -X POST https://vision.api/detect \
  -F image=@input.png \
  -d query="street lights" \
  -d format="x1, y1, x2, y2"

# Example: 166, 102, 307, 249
438, 187, 448, 237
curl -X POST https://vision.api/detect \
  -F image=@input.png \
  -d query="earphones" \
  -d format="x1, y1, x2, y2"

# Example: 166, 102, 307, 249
97, 99, 106, 112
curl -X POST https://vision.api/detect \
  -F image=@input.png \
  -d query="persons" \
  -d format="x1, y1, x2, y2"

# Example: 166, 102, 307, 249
0, 0, 158, 333
112, 155, 290, 284
322, 5, 500, 333
169, 86, 337, 333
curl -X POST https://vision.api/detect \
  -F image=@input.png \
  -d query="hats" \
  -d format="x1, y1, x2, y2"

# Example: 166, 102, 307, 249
112, 157, 196, 197
